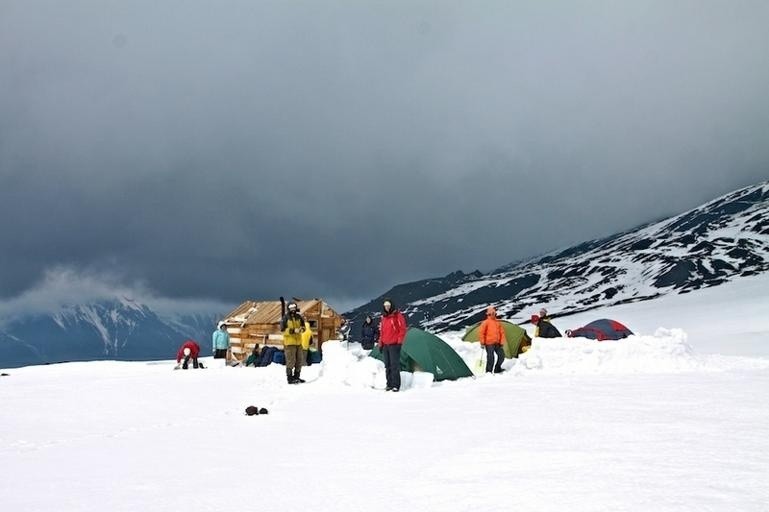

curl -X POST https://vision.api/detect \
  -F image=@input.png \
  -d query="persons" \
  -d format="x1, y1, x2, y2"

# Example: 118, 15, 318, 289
279, 301, 305, 383
176, 340, 200, 369
378, 298, 407, 392
337, 317, 351, 340
535, 308, 551, 337
211, 321, 231, 359
479, 306, 505, 373
532, 315, 562, 338
301, 322, 314, 366
361, 315, 380, 350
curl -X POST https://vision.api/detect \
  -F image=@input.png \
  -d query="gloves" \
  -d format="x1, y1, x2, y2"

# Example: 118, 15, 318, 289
379, 347, 382, 353
396, 346, 401, 352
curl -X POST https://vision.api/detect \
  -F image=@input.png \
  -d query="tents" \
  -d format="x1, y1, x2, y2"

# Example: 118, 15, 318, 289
565, 319, 635, 340
368, 319, 474, 381
461, 319, 533, 359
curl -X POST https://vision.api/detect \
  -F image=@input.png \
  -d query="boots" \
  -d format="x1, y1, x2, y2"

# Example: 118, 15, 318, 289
287, 372, 305, 384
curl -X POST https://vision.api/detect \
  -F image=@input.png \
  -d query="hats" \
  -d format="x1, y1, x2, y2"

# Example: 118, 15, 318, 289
289, 303, 297, 311
532, 315, 540, 323
540, 308, 547, 314
